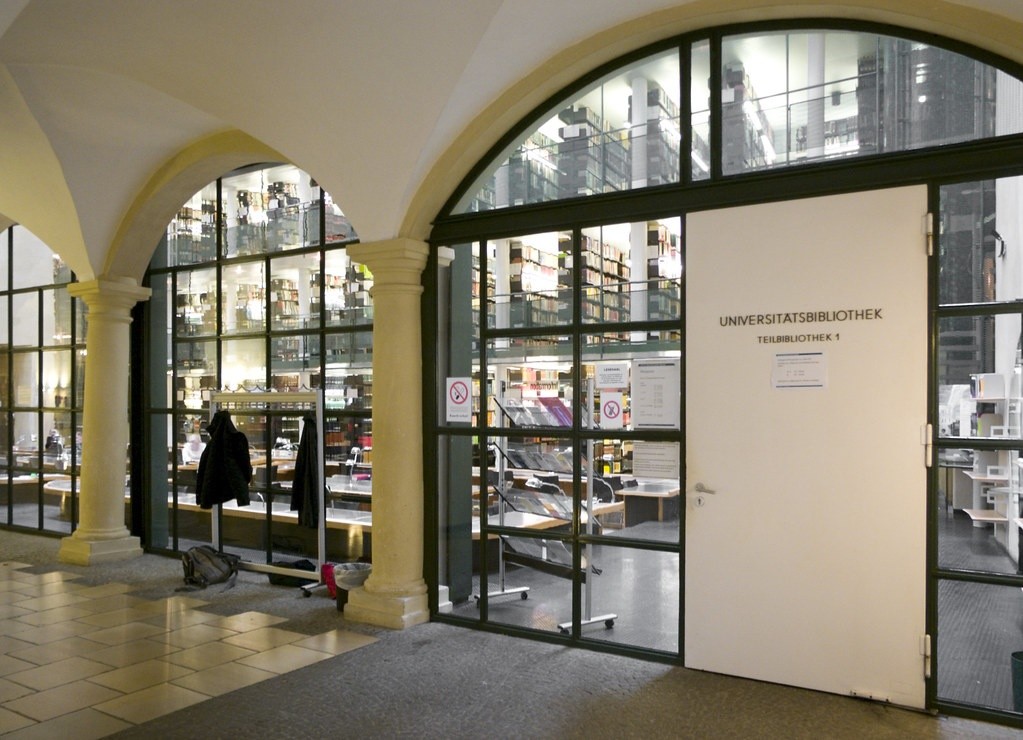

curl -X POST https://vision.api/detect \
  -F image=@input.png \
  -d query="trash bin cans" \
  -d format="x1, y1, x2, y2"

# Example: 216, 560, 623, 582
333, 562, 372, 612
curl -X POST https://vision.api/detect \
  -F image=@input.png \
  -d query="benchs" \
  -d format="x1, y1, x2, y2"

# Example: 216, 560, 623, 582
45, 479, 372, 564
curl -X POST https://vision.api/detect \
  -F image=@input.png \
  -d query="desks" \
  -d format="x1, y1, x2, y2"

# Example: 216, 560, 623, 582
620, 479, 680, 522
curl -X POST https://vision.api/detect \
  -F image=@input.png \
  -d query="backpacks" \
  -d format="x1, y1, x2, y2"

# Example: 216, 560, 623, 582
174, 545, 241, 593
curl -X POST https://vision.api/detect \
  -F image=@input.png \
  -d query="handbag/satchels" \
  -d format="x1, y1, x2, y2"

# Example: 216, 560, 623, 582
321, 559, 341, 599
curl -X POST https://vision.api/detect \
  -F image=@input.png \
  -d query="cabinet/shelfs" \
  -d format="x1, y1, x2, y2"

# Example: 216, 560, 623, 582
163, 38, 1023, 575
